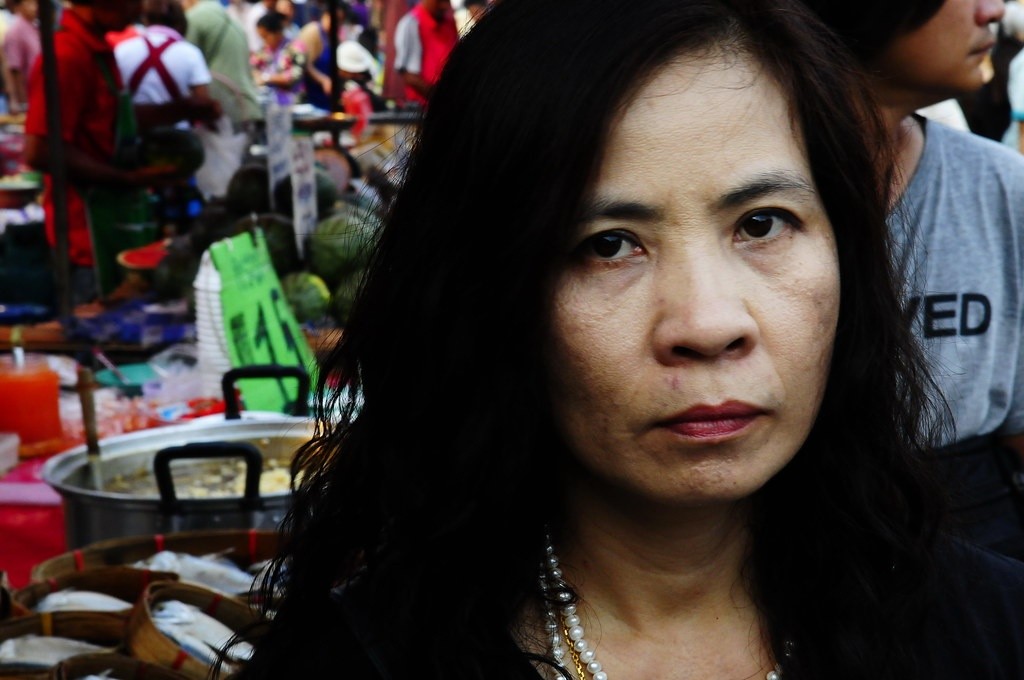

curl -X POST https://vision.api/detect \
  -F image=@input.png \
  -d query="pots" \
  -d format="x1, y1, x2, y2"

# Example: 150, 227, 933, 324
40, 364, 354, 552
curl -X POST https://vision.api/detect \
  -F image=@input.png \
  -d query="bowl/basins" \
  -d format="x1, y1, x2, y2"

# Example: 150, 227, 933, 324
192, 250, 239, 401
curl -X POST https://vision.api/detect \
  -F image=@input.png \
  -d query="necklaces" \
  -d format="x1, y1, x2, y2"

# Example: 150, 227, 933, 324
539, 541, 793, 679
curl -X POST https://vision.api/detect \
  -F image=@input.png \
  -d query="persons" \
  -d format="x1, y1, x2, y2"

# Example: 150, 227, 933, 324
183, 1, 261, 120
803, 0, 1024, 562
298, 2, 346, 114
393, 0, 460, 107
113, 0, 224, 233
218, 0, 1024, 680
25, 0, 223, 312
252, 11, 305, 105
0, 0, 41, 115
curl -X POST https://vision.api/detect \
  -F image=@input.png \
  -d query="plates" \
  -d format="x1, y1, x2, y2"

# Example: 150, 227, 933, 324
94, 363, 160, 393
